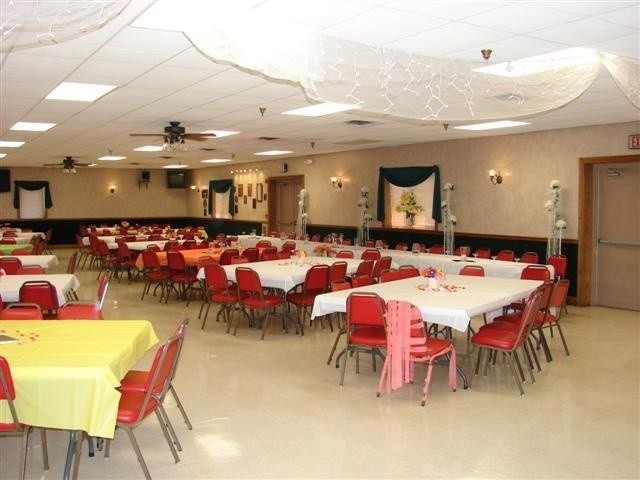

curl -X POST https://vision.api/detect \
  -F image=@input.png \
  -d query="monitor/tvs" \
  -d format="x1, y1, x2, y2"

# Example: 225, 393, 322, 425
168, 171, 185, 185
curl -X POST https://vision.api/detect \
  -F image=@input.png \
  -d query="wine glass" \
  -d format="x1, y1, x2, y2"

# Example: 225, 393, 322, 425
445, 250, 521, 265
208, 229, 433, 267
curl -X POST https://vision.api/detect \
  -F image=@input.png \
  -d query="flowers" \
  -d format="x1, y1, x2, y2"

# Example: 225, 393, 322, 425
395, 190, 425, 214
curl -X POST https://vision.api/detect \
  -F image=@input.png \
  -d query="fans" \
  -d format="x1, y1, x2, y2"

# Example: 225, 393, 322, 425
43, 156, 96, 168
129, 122, 217, 141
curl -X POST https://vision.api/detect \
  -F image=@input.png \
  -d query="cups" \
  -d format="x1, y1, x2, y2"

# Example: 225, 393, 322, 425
113, 222, 200, 245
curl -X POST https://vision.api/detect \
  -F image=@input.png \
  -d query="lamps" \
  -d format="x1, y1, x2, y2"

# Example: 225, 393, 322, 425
62, 168, 77, 176
187, 186, 199, 192
489, 170, 502, 185
163, 137, 188, 152
331, 177, 342, 188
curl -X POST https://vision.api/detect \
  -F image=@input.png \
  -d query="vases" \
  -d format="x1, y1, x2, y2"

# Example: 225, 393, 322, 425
406, 212, 416, 226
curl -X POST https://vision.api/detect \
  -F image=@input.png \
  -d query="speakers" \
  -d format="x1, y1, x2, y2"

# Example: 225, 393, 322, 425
0, 169, 10, 192
143, 172, 149, 179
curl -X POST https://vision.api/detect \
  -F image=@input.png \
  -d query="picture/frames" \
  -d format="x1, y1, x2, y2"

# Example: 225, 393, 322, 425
235, 183, 263, 213
